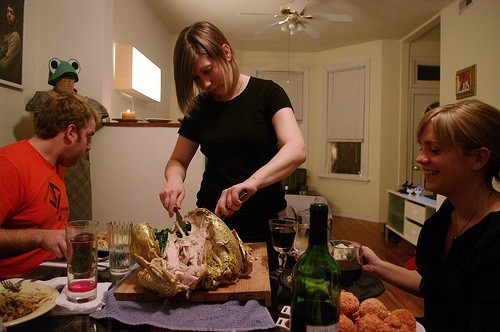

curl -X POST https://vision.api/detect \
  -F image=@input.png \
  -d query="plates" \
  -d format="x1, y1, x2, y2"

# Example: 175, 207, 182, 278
97, 249, 110, 257
279, 267, 385, 301
178, 119, 184, 122
144, 118, 172, 123
0, 280, 60, 327
415, 321, 426, 332
113, 118, 143, 122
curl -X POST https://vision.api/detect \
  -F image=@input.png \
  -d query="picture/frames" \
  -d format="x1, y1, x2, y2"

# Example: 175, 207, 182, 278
456, 63, 478, 99
0, 0, 25, 92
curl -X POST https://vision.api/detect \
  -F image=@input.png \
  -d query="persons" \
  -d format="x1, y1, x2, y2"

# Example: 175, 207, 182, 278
159, 21, 307, 269
0, 92, 97, 281
349, 100, 500, 332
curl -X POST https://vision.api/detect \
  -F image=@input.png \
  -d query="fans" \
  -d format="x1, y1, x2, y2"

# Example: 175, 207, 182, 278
240, 0, 355, 39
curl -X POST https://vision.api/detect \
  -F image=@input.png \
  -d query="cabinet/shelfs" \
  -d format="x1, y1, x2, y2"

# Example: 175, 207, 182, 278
385, 189, 437, 246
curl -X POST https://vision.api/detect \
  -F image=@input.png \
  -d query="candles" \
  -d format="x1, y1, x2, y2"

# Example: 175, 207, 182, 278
122, 109, 136, 119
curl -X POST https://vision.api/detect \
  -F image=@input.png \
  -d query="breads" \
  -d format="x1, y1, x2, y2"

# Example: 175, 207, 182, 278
339, 292, 417, 332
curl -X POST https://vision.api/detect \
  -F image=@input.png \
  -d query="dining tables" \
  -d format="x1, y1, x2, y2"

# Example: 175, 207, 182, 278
7, 240, 293, 332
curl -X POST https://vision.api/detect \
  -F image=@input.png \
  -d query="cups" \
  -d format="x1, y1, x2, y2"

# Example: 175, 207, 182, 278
106, 220, 133, 275
294, 223, 309, 255
327, 240, 363, 291
65, 219, 99, 303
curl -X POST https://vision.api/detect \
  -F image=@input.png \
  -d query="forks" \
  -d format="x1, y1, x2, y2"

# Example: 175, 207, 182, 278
1, 270, 43, 291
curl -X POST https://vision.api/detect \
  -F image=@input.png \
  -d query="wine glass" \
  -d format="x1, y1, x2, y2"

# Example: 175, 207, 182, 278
268, 219, 297, 279
414, 188, 422, 196
407, 188, 414, 196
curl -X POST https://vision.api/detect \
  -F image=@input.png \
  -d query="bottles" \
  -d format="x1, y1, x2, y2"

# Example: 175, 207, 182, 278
290, 203, 341, 331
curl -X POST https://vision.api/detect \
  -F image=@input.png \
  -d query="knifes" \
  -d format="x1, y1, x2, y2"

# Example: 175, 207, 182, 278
173, 207, 186, 227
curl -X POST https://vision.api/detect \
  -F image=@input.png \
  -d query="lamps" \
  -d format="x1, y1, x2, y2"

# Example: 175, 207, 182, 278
114, 44, 162, 103
278, 13, 313, 84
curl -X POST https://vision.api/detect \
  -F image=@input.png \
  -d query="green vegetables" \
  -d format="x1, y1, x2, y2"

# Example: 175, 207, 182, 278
154, 223, 190, 251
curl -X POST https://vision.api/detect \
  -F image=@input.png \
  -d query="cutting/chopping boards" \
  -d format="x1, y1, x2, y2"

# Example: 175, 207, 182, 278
114, 240, 271, 302
